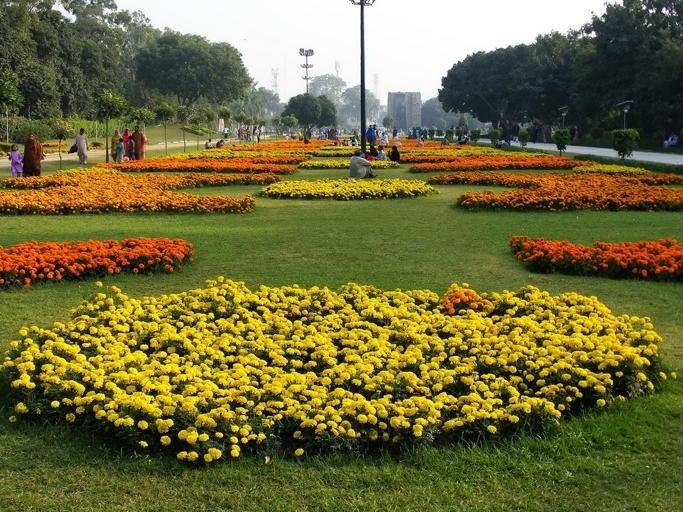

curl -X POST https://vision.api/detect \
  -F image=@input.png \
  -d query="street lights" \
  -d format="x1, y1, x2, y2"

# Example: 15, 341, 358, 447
299, 48, 314, 93
615, 99, 633, 161
558, 105, 568, 153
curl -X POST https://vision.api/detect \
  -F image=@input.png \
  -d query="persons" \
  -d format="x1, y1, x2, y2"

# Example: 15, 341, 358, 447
220, 123, 262, 143
6, 142, 23, 178
126, 135, 134, 160
439, 135, 449, 146
204, 138, 213, 149
663, 129, 680, 148
215, 139, 225, 149
132, 124, 147, 160
454, 135, 468, 145
110, 128, 122, 162
115, 137, 123, 162
289, 123, 389, 149
414, 136, 424, 147
21, 131, 46, 176
372, 145, 387, 161
366, 144, 378, 161
394, 137, 401, 148
386, 145, 400, 162
494, 140, 502, 148
74, 127, 90, 165
501, 139, 509, 148
121, 127, 132, 157
348, 150, 378, 179
